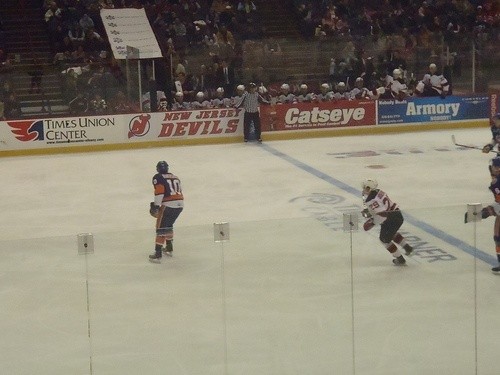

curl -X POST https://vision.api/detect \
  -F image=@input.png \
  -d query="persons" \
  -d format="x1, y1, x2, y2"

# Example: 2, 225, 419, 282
481, 113, 500, 272
234, 82, 270, 143
39, 0, 294, 114
360, 179, 412, 265
148, 161, 183, 263
0, 45, 19, 119
289, 0, 500, 103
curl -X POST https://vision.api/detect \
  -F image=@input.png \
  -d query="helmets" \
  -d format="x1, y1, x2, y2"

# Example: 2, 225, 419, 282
393, 69, 401, 75
237, 85, 246, 90
217, 87, 224, 93
362, 177, 378, 196
429, 63, 437, 68
156, 161, 169, 174
196, 91, 204, 98
492, 113, 500, 121
259, 77, 364, 95
175, 91, 182, 96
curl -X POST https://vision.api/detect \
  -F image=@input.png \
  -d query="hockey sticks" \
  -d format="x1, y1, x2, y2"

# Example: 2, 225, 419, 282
452, 134, 500, 154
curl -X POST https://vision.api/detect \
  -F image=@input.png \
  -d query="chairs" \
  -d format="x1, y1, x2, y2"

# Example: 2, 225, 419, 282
322, 36, 419, 68
176, 40, 269, 84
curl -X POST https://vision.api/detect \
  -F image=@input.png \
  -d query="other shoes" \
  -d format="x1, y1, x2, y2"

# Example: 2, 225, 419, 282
491, 266, 500, 272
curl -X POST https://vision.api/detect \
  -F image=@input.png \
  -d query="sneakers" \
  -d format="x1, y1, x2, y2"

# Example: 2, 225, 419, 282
243, 138, 248, 142
403, 243, 414, 256
162, 240, 173, 253
392, 255, 406, 265
257, 137, 262, 142
149, 249, 162, 259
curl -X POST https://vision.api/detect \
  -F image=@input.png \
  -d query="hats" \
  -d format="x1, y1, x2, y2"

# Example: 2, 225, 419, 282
247, 81, 258, 88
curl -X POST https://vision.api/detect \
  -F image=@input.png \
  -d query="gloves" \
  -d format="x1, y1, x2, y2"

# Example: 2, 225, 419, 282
480, 207, 490, 220
150, 202, 161, 218
482, 144, 493, 153
